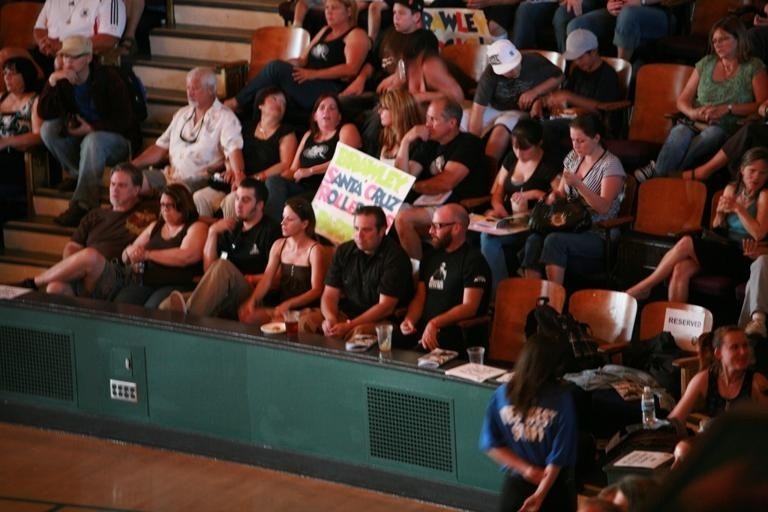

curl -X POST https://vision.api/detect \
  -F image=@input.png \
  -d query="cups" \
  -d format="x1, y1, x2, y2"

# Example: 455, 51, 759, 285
511, 198, 527, 217
375, 325, 394, 352
281, 311, 300, 337
467, 347, 485, 374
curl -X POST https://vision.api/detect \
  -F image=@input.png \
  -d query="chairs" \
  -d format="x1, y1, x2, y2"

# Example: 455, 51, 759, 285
488, 278, 567, 368
520, 50, 567, 75
691, 0, 743, 57
596, 176, 637, 278
0, 0, 44, 52
566, 288, 637, 365
440, 41, 490, 88
620, 175, 708, 251
735, 279, 749, 304
570, 58, 632, 160
596, 301, 714, 402
0, 47, 46, 98
691, 190, 741, 293
629, 63, 694, 157
246, 27, 310, 86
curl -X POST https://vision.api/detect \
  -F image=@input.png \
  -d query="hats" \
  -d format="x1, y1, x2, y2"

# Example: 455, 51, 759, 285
56, 36, 93, 57
562, 29, 598, 60
396, 0, 424, 12
487, 39, 522, 75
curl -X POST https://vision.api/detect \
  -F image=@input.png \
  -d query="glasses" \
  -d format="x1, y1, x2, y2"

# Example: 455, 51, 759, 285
179, 118, 203, 144
431, 222, 455, 229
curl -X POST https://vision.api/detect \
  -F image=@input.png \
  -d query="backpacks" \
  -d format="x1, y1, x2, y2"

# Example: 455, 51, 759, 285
100, 65, 147, 122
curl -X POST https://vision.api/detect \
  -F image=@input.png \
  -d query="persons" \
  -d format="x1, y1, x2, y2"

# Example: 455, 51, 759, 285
20, 1, 694, 299
398, 203, 492, 360
236, 197, 328, 326
160, 176, 281, 318
634, 17, 768, 185
112, 182, 208, 309
296, 206, 416, 345
0, 57, 42, 222
478, 304, 586, 512
668, 100, 768, 181
624, 145, 768, 304
667, 324, 768, 435
736, 238, 768, 340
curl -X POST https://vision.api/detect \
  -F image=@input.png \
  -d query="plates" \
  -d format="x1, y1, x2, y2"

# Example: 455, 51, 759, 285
260, 323, 286, 334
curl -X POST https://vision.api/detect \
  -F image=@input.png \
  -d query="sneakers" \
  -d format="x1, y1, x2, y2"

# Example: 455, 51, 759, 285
170, 291, 186, 314
744, 319, 766, 339
56, 205, 84, 226
635, 161, 654, 182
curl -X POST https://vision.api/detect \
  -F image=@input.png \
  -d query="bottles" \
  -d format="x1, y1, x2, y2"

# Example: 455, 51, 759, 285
132, 261, 145, 287
642, 387, 656, 428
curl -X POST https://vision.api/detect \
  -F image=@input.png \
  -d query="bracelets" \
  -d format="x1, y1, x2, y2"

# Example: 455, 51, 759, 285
727, 103, 732, 113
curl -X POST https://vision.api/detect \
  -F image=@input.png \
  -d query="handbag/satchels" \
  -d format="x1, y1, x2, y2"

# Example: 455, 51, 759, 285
529, 199, 591, 236
524, 296, 574, 376
